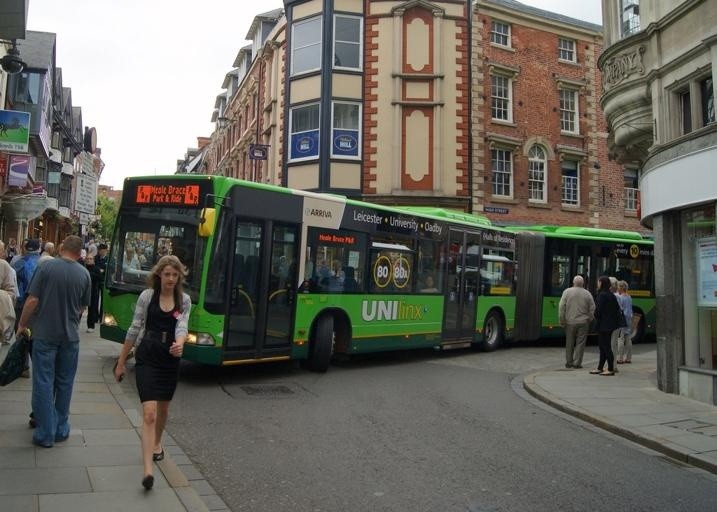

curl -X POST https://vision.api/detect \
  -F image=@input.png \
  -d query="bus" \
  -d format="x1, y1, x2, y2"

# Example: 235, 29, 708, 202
100, 174, 655, 375
455, 243, 515, 288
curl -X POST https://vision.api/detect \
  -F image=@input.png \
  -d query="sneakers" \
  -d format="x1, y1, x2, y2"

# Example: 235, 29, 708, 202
590, 369, 603, 374
600, 371, 615, 375
87, 328, 94, 332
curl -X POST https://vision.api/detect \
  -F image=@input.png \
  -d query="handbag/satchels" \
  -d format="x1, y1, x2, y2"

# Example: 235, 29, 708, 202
0, 332, 28, 387
619, 310, 628, 328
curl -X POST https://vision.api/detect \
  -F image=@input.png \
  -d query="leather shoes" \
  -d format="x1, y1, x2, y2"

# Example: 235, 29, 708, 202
142, 476, 154, 489
153, 448, 164, 460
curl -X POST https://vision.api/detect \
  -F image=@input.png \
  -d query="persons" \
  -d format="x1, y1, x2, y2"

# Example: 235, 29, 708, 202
420, 274, 439, 294
594, 276, 617, 375
0, 234, 154, 448
607, 277, 622, 373
617, 280, 632, 363
559, 275, 596, 368
115, 255, 192, 488
318, 259, 346, 292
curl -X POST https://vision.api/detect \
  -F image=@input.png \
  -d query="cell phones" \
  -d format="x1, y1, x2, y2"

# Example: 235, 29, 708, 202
119, 374, 123, 383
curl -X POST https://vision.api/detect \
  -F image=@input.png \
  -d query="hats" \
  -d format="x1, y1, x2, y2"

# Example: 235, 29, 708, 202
97, 244, 108, 248
25, 239, 39, 251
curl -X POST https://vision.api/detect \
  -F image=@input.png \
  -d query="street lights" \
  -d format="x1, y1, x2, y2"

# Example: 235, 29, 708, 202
0, 38, 27, 76
